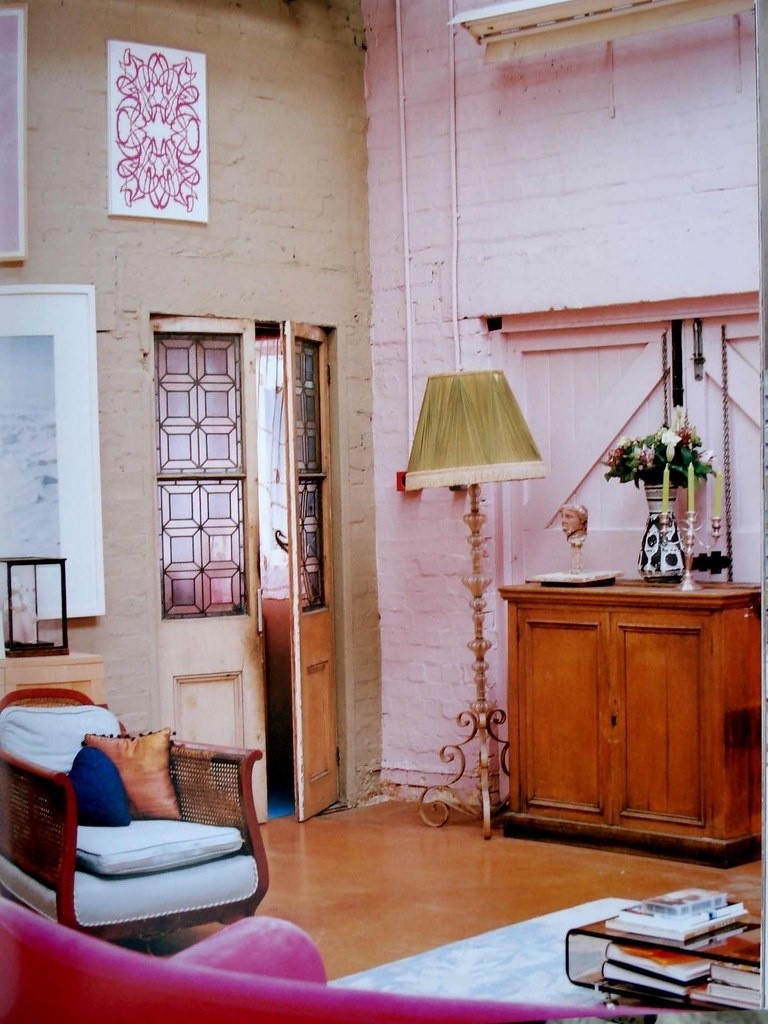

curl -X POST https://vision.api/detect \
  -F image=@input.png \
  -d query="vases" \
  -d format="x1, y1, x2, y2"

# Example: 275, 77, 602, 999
636, 483, 687, 584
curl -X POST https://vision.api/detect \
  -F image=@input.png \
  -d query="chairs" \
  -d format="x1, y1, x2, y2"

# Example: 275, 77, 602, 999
0, 687, 270, 913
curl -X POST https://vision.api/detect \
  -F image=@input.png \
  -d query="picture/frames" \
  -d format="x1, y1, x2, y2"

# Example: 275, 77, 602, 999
0, 2, 29, 262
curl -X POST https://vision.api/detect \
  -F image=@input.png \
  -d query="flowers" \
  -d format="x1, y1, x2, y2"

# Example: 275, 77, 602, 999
598, 404, 717, 490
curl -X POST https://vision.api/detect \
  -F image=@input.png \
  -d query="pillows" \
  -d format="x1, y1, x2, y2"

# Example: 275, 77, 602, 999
81, 727, 184, 821
67, 746, 130, 827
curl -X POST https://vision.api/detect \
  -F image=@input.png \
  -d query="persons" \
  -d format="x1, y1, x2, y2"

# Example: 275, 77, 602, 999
560, 504, 588, 547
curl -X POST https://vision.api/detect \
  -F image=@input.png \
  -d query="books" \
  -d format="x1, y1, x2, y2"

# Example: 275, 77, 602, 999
601, 887, 761, 1011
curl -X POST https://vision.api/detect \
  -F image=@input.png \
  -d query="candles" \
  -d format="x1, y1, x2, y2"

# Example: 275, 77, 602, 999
662, 463, 669, 513
687, 462, 695, 511
712, 467, 723, 518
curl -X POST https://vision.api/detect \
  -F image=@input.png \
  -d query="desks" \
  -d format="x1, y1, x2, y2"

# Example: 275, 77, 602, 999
327, 899, 640, 1006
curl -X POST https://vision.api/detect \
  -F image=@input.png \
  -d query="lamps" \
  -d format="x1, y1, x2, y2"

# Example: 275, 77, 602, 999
403, 370, 546, 839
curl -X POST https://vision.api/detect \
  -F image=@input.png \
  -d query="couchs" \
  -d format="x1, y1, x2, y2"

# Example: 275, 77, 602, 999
0, 899, 702, 1024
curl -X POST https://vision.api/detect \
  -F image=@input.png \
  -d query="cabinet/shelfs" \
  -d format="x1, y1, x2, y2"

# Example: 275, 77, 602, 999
498, 578, 763, 870
